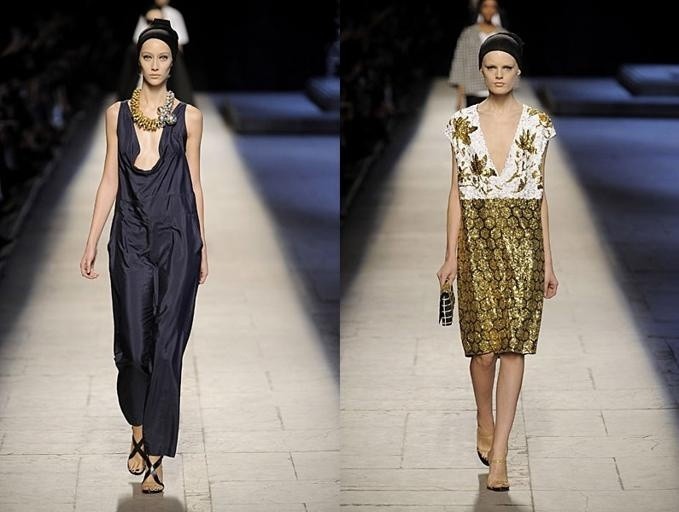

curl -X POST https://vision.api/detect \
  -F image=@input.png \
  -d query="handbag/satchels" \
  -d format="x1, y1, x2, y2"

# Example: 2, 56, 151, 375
438, 281, 456, 326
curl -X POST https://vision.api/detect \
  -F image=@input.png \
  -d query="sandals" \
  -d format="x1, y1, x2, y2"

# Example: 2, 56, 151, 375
127, 435, 147, 476
476, 429, 491, 466
486, 457, 510, 491
141, 454, 165, 494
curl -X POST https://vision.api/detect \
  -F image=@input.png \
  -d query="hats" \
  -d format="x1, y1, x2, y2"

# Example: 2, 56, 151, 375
478, 31, 526, 72
135, 19, 179, 64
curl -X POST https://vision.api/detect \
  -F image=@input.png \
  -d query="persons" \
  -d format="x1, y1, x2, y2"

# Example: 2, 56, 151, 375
80, 18, 216, 493
448, 0, 507, 111
340, 24, 436, 221
0, 66, 104, 260
130, 0, 189, 83
436, 29, 559, 490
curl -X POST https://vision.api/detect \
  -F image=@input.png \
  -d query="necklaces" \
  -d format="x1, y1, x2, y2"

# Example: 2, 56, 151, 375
128, 87, 178, 132
